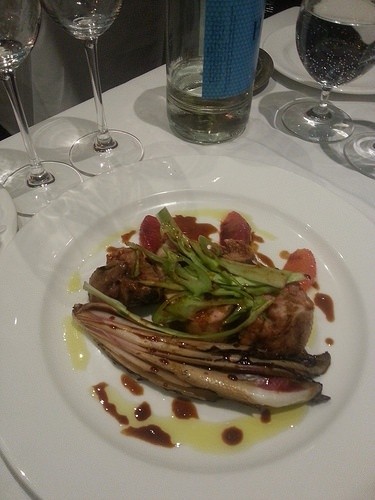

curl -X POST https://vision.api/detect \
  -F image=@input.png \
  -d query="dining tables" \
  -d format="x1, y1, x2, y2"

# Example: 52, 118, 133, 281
0, 0, 375, 499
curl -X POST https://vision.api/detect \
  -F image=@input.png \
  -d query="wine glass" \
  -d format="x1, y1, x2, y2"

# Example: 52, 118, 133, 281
344, 132, 375, 179
40, 0, 144, 176
0, 0, 83, 216
282, 0, 375, 143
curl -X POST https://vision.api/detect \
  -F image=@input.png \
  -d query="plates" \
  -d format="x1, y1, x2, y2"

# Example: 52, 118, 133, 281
0, 156, 375, 500
263, 24, 375, 94
253, 48, 275, 96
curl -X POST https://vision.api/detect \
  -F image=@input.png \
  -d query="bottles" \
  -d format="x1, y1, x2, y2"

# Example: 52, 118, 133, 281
165, 0, 266, 145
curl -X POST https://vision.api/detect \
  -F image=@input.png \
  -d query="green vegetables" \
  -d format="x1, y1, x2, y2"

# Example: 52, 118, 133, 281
83, 207, 306, 342
